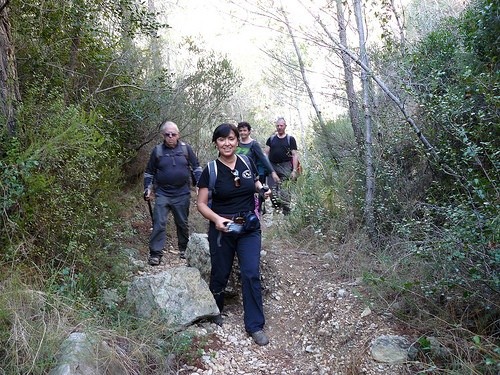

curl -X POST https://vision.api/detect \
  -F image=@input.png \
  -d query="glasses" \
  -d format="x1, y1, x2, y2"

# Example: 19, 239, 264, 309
231, 169, 240, 187
163, 132, 179, 136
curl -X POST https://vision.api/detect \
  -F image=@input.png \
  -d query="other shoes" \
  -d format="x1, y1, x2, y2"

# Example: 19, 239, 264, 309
180, 250, 185, 259
148, 255, 162, 266
211, 314, 223, 327
250, 329, 270, 346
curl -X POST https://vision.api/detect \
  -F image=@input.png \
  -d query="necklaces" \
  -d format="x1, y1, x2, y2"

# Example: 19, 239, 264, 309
221, 155, 236, 163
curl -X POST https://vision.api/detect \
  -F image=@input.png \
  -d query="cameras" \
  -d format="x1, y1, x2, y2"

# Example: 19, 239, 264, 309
226, 222, 243, 234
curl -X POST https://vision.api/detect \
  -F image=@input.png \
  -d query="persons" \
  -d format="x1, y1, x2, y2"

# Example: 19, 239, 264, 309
265, 118, 298, 216
143, 122, 203, 265
234, 121, 280, 213
197, 124, 271, 346
